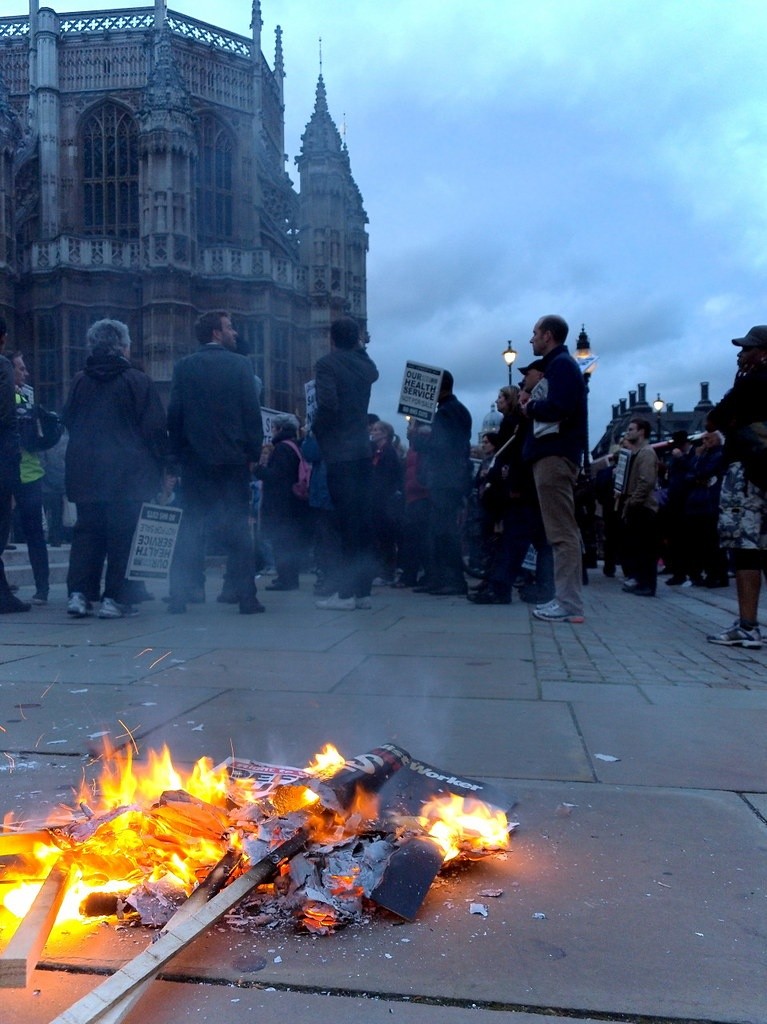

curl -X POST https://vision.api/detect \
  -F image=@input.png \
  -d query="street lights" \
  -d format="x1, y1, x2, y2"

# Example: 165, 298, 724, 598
502, 340, 517, 386
572, 323, 599, 476
653, 394, 666, 441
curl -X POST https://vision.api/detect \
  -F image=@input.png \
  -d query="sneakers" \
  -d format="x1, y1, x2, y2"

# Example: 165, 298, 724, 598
706, 618, 764, 648
68, 592, 95, 616
100, 595, 140, 618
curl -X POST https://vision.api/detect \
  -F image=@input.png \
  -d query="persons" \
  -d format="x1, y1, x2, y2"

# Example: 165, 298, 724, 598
5, 350, 56, 608
704, 324, 767, 650
520, 314, 591, 622
311, 317, 380, 612
57, 320, 168, 620
0, 319, 31, 615
164, 310, 266, 615
0, 359, 732, 618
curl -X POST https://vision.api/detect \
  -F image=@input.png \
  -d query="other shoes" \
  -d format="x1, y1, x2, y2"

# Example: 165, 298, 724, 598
465, 580, 514, 604
412, 577, 469, 596
620, 584, 658, 597
664, 576, 689, 585
0, 597, 32, 615
30, 593, 47, 606
166, 595, 190, 612
312, 592, 358, 611
237, 595, 268, 612
354, 596, 373, 611
160, 586, 208, 604
263, 577, 302, 591
218, 585, 257, 603
530, 597, 584, 624
385, 575, 420, 588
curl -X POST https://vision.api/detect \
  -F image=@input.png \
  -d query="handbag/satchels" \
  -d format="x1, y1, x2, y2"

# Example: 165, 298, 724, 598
63, 430, 98, 504
15, 401, 64, 452
573, 477, 598, 523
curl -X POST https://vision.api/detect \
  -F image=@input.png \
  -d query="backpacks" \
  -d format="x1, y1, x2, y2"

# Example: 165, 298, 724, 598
283, 439, 311, 501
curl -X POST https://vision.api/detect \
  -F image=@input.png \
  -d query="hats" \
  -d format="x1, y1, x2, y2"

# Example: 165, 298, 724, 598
668, 430, 694, 446
517, 358, 544, 376
732, 326, 767, 347
